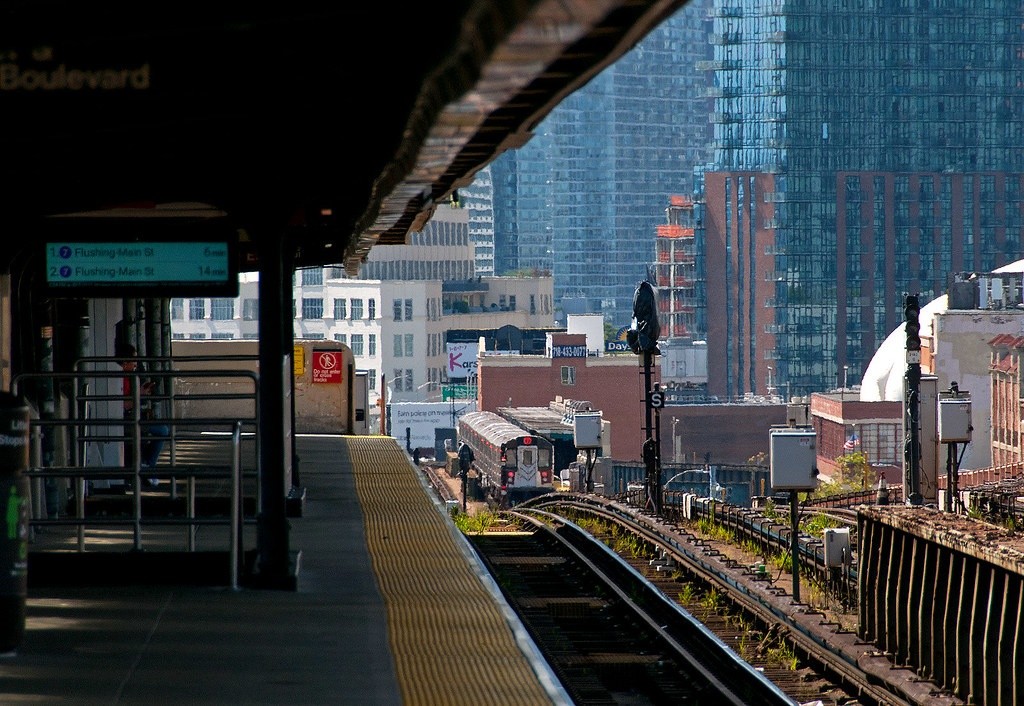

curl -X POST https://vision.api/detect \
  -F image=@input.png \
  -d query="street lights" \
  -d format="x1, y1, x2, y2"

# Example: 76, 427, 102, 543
671, 419, 679, 463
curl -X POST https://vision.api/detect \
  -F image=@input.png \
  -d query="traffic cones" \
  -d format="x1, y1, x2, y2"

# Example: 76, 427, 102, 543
876, 470, 890, 506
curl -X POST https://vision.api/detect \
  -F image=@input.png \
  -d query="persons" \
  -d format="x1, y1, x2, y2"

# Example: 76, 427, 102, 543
115, 342, 168, 495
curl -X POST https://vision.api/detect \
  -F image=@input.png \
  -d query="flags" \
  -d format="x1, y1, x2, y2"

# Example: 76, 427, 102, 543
844, 435, 861, 450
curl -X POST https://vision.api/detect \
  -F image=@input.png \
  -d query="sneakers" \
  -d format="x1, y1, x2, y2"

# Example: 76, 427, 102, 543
141, 464, 159, 486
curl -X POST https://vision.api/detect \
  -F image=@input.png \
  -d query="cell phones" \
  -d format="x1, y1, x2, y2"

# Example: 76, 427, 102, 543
143, 382, 151, 389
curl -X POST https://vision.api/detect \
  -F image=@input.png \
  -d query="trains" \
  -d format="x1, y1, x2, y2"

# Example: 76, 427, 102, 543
456, 410, 555, 507
